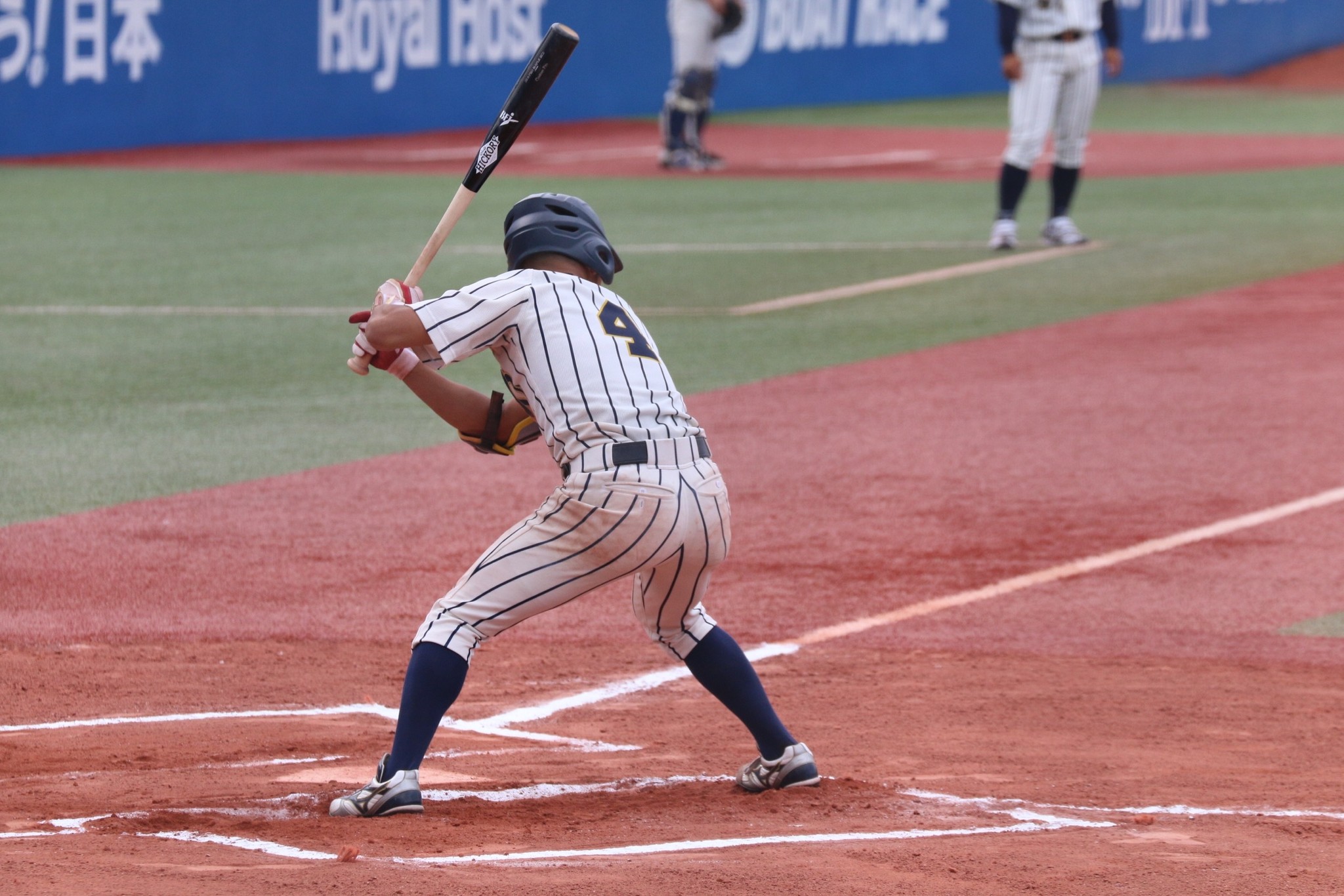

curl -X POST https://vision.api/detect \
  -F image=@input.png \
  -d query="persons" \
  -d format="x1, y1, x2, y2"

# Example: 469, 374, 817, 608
329, 193, 823, 817
663, 0, 744, 169
990, 0, 1123, 248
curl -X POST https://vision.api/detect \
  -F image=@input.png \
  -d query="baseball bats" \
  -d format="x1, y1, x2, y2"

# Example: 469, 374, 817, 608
347, 20, 578, 379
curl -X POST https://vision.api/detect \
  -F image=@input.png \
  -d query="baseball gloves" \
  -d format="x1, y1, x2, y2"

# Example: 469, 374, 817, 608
712, 1, 743, 39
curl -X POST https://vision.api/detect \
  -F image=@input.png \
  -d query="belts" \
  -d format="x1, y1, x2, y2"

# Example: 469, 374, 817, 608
1048, 29, 1084, 44
561, 435, 709, 480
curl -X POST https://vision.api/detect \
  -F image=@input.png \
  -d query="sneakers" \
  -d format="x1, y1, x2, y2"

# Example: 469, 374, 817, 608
327, 753, 425, 817
1039, 215, 1088, 249
988, 218, 1025, 251
733, 742, 828, 792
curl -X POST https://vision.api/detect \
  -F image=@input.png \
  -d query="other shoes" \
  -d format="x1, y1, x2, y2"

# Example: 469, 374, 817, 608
700, 149, 730, 173
655, 147, 707, 171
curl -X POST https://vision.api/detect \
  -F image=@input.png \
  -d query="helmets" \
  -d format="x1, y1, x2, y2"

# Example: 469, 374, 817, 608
500, 190, 632, 282
